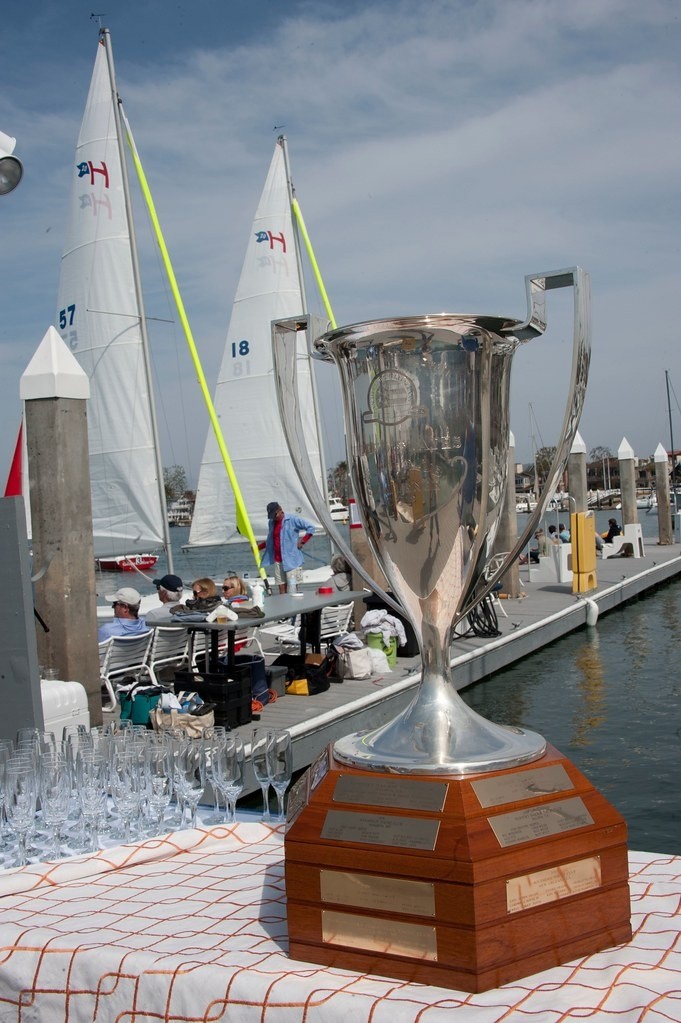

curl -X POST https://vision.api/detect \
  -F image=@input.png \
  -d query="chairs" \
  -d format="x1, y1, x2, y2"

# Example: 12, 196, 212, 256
98, 599, 355, 714
484, 551, 513, 618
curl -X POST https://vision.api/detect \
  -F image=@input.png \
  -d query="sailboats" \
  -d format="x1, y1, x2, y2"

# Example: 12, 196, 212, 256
177, 121, 342, 595
514, 370, 568, 514
52, 13, 274, 624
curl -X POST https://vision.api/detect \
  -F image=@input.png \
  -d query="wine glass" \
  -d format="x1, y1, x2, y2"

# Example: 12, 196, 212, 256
0, 720, 292, 874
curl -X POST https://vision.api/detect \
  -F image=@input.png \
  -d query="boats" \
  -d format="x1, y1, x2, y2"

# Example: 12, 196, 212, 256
329, 496, 349, 522
613, 370, 681, 531
94, 551, 161, 573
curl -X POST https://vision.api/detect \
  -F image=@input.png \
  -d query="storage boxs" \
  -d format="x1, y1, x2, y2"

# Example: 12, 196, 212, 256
39, 678, 90, 760
173, 666, 253, 731
361, 591, 420, 658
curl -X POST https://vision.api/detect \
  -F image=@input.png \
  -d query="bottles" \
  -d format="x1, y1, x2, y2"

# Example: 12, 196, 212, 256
253, 583, 264, 607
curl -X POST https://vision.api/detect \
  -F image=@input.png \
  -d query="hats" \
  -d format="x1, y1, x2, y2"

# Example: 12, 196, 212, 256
153, 574, 183, 593
105, 587, 141, 605
534, 528, 544, 539
267, 501, 278, 518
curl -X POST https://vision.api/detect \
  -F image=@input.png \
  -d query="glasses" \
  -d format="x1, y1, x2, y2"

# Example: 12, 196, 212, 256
111, 602, 125, 608
156, 585, 163, 591
193, 590, 204, 596
221, 585, 234, 592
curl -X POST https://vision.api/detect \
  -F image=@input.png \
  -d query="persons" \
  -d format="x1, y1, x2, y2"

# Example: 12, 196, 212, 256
519, 519, 623, 565
98, 575, 251, 694
317, 555, 354, 593
252, 501, 316, 624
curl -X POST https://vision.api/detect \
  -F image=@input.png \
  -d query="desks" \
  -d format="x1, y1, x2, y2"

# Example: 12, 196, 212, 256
144, 591, 374, 676
0, 803, 681, 1023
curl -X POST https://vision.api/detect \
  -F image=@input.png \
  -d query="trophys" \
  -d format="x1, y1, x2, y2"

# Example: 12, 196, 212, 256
271, 264, 633, 993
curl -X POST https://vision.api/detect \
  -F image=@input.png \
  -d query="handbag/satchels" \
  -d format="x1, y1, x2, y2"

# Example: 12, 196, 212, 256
269, 653, 330, 697
325, 643, 346, 683
115, 651, 276, 740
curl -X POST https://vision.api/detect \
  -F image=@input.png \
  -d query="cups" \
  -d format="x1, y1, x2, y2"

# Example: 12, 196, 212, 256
279, 583, 286, 594
216, 605, 229, 625
